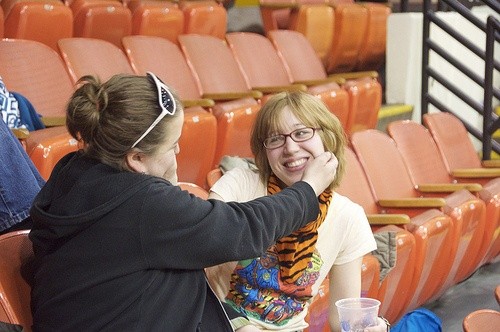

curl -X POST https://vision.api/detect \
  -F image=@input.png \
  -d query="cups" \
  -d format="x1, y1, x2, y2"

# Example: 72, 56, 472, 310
335, 298, 381, 332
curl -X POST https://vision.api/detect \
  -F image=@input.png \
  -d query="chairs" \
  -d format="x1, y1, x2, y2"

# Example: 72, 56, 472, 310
0, 0, 500, 331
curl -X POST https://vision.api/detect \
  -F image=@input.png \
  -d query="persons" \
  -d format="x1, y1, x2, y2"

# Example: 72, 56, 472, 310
28, 70, 339, 332
207, 90, 378, 332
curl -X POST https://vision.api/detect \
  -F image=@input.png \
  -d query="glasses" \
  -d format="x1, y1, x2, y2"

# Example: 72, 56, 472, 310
130, 72, 177, 149
262, 126, 323, 150
